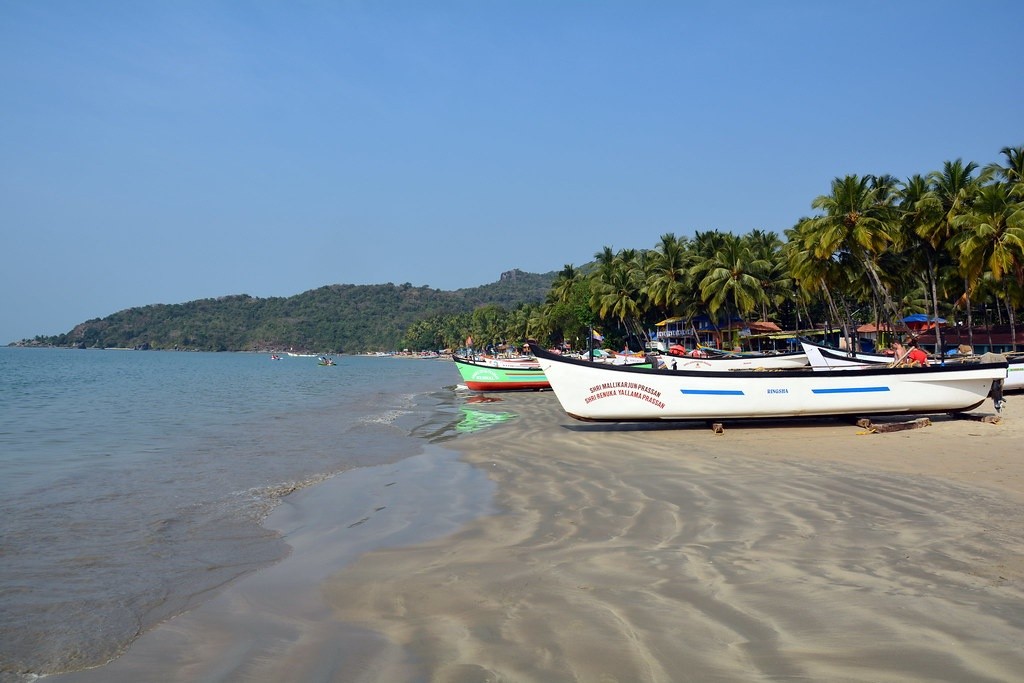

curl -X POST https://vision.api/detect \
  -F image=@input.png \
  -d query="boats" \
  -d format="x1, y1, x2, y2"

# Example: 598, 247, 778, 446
643, 348, 808, 371
318, 362, 336, 366
527, 343, 1008, 422
451, 355, 652, 390
270, 357, 283, 360
800, 342, 1024, 390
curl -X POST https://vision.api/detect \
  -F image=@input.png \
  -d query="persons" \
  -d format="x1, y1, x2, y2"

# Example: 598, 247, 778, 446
887, 338, 927, 368
323, 357, 333, 364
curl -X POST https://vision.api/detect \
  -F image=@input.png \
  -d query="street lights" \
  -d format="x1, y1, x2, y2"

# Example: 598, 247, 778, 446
793, 294, 799, 352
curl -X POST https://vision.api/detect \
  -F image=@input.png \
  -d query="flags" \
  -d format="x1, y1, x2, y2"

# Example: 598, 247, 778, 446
593, 330, 602, 342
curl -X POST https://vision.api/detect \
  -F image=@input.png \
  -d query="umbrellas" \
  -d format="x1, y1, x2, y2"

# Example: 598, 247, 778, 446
896, 313, 949, 324
